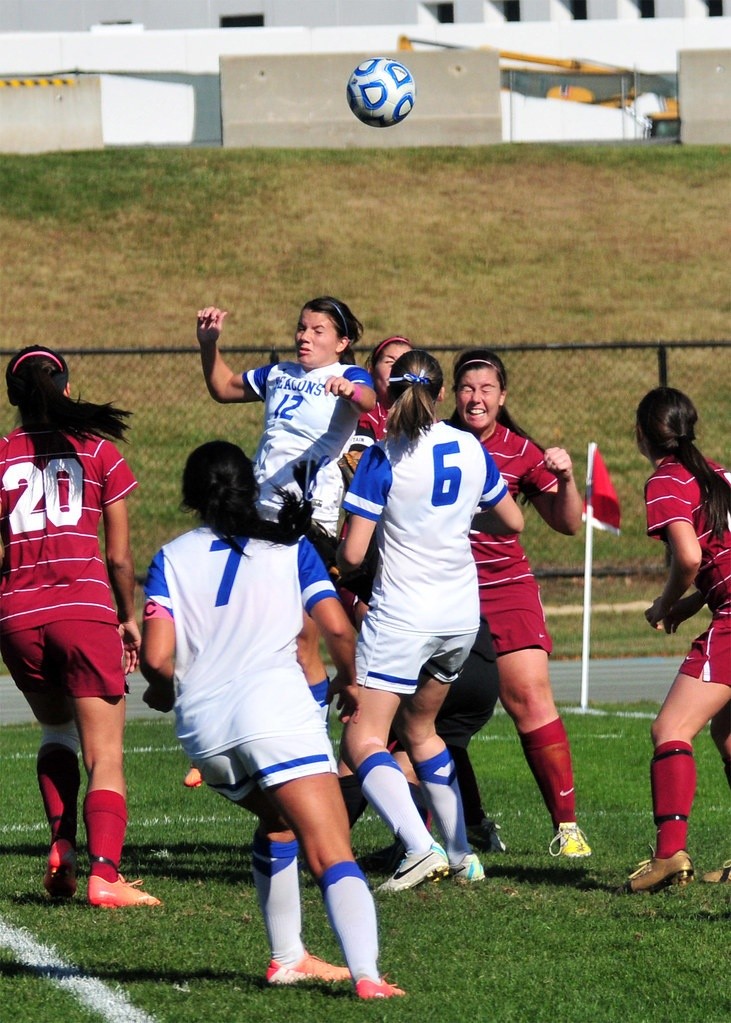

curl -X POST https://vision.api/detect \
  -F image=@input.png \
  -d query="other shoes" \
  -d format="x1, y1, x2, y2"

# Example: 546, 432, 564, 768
184, 767, 204, 786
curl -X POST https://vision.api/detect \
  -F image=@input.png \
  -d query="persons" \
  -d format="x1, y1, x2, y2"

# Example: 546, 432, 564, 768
0, 344, 163, 908
624, 387, 731, 895
138, 440, 408, 999
196, 295, 592, 893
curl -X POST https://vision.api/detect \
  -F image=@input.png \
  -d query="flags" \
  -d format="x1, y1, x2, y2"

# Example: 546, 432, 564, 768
583, 444, 622, 534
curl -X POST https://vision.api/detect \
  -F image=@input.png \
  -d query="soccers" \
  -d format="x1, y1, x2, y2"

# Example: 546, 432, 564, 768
346, 56, 418, 127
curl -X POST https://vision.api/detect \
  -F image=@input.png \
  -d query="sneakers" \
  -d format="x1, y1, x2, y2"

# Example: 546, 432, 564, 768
355, 972, 405, 998
376, 841, 450, 893
618, 843, 694, 894
548, 821, 591, 858
43, 840, 76, 897
88, 874, 161, 908
267, 949, 354, 984
465, 818, 505, 852
701, 859, 731, 882
446, 854, 485, 885
